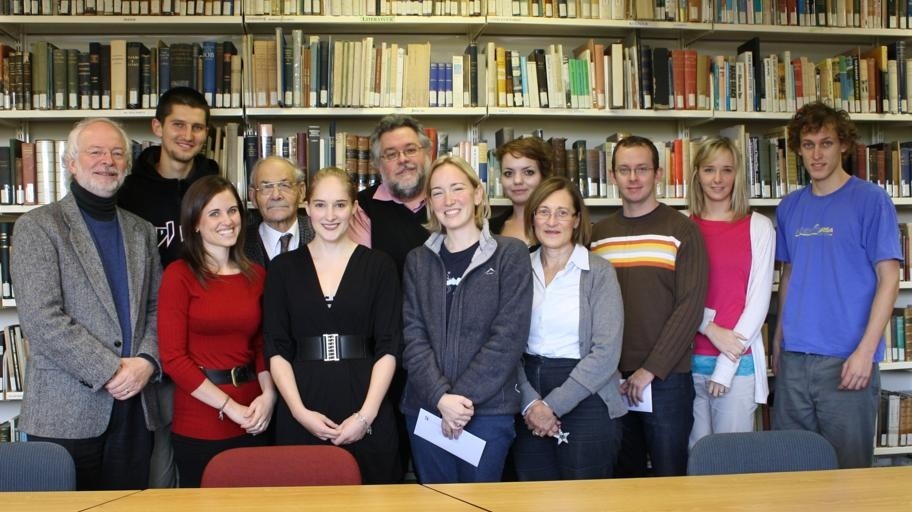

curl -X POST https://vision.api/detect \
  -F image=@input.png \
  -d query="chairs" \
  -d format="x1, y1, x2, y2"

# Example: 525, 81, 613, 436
688, 429, 838, 476
201, 446, 362, 488
0, 439, 78, 492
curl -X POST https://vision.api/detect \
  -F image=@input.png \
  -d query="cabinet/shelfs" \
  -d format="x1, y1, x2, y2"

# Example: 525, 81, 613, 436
246, 0, 487, 483
714, 0, 912, 467
487, 0, 714, 477
1, 0, 246, 442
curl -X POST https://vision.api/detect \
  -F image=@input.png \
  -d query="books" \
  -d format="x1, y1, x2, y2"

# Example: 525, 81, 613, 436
757, 223, 912, 465
1, 0, 911, 197
0, 224, 29, 446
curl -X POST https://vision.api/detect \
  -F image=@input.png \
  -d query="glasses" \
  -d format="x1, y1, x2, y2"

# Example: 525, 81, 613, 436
380, 146, 424, 161
614, 166, 654, 176
533, 208, 577, 221
255, 181, 298, 192
76, 145, 127, 159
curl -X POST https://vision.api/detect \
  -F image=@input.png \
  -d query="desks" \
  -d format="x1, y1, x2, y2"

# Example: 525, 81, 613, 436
2, 488, 146, 512
79, 484, 490, 512
419, 466, 912, 512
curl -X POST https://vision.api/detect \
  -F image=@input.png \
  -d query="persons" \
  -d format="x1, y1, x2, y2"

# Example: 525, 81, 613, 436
7, 84, 778, 491
769, 101, 905, 470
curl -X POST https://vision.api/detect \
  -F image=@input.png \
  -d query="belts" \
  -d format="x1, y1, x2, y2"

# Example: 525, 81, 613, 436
199, 364, 257, 388
296, 333, 372, 363
